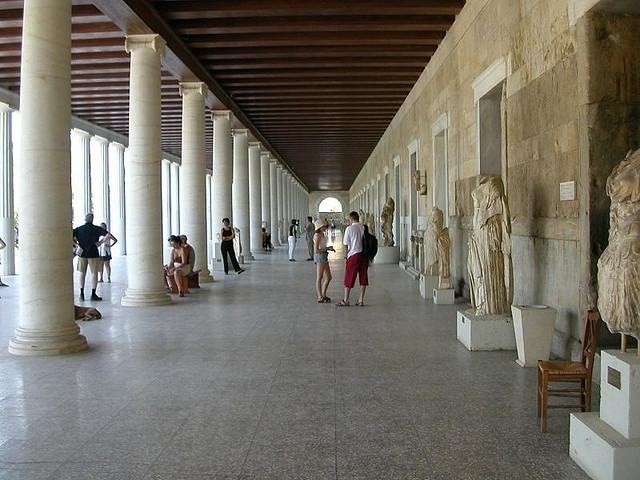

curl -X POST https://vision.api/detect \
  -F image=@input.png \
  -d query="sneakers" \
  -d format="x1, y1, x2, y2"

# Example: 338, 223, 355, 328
91, 294, 102, 300
79, 294, 83, 300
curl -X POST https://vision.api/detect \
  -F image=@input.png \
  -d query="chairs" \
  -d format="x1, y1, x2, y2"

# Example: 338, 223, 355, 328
536, 310, 602, 434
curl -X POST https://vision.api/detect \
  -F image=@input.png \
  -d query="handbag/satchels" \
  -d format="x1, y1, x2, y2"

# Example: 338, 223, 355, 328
362, 231, 378, 261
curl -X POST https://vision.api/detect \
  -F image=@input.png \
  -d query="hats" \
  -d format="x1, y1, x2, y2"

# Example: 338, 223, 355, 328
315, 219, 327, 230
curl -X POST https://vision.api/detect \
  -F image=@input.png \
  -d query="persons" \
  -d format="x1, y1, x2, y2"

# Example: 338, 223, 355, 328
70, 213, 112, 301
166, 216, 336, 296
97, 222, 118, 284
313, 219, 335, 303
0, 238, 10, 286
332, 212, 372, 306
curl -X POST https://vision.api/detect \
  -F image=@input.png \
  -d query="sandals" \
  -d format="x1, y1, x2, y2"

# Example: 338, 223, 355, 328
318, 296, 330, 303
356, 300, 363, 306
336, 300, 351, 306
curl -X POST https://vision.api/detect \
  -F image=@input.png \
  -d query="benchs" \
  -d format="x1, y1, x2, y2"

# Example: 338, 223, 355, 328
165, 268, 201, 293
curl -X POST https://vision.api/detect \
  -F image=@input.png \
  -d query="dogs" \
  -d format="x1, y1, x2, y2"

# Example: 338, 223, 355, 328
74, 305, 101, 321
